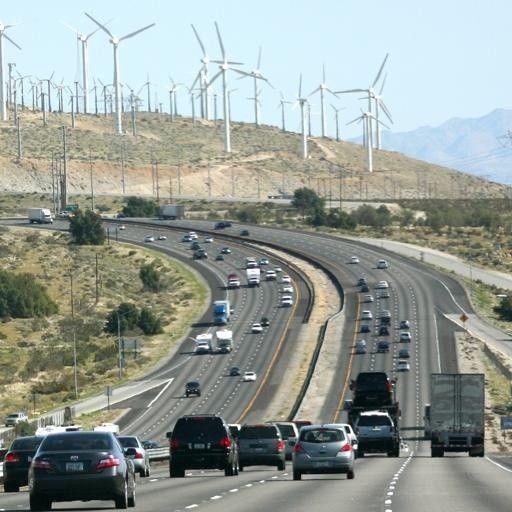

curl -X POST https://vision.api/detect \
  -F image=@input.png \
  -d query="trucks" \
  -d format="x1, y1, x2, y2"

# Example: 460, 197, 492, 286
194, 300, 234, 353
158, 204, 184, 220
29, 207, 53, 224
228, 257, 260, 288
423, 373, 485, 456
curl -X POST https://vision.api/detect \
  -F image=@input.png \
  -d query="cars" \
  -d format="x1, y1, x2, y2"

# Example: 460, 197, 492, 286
252, 258, 294, 333
0, 413, 152, 510
167, 373, 402, 480
352, 256, 412, 371
55, 211, 69, 218
119, 221, 249, 260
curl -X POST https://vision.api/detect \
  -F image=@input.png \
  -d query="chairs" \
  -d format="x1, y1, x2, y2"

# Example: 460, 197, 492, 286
304, 434, 337, 441
55, 440, 108, 449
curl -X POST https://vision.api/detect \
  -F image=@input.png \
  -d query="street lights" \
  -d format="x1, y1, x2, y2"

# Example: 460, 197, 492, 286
117, 306, 141, 378
